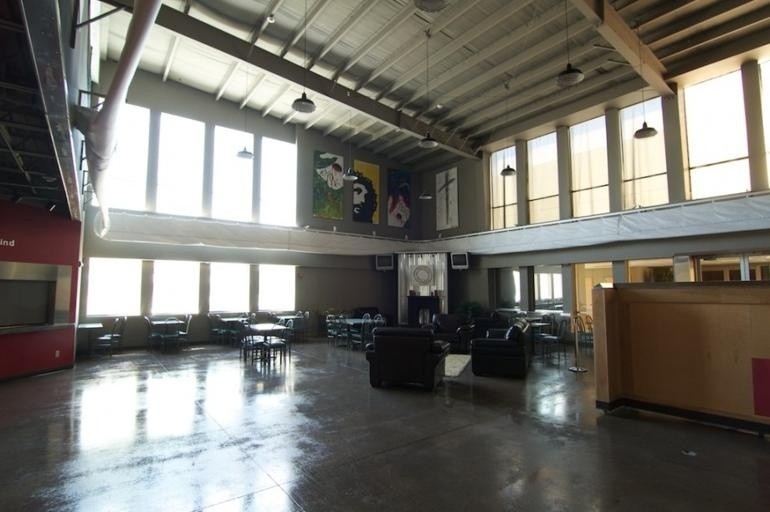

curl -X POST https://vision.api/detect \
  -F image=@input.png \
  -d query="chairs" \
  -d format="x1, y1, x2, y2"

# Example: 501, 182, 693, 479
324, 307, 573, 391
97, 310, 310, 367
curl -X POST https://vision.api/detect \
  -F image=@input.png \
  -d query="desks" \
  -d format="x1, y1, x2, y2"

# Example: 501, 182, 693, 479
77, 322, 104, 356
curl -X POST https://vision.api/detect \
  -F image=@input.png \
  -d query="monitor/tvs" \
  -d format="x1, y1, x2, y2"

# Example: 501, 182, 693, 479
376, 254, 394, 270
450, 252, 469, 270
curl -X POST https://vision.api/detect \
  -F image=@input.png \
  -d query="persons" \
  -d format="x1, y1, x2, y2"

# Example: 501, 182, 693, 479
352, 172, 377, 221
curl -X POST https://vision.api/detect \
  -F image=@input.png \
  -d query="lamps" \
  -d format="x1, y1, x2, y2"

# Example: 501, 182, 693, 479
558, 0, 585, 86
292, 0, 316, 114
417, 37, 438, 149
500, 88, 517, 175
342, 109, 358, 181
633, 19, 658, 138
